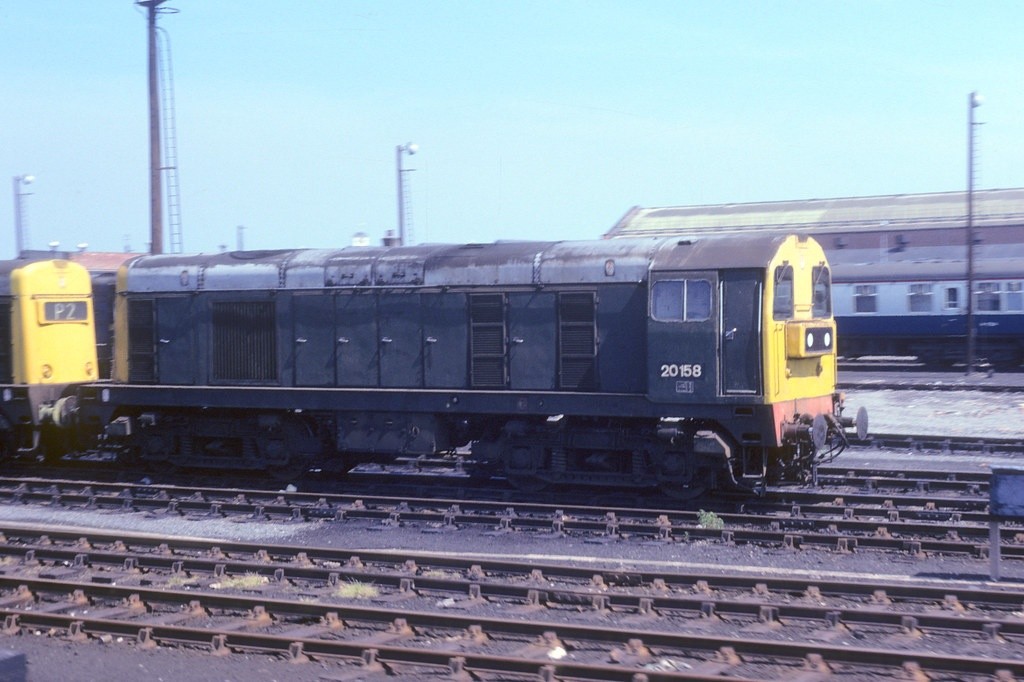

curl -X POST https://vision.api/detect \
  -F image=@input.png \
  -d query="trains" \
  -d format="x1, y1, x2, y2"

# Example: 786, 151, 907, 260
823, 245, 1024, 374
0, 225, 870, 503
